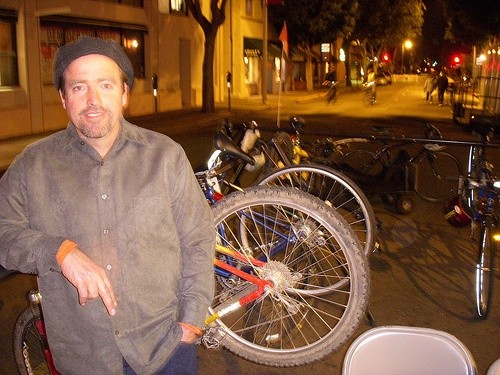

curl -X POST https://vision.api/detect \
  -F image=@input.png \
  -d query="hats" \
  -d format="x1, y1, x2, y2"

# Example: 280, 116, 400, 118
53, 37, 134, 89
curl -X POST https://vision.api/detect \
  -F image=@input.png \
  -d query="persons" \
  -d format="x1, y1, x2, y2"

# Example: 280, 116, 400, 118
423, 74, 436, 105
434, 71, 448, 107
362, 68, 376, 93
0, 35, 216, 375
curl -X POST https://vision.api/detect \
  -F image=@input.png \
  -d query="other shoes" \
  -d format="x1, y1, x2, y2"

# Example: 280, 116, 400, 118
438, 103, 443, 106
429, 101, 433, 104
425, 101, 428, 104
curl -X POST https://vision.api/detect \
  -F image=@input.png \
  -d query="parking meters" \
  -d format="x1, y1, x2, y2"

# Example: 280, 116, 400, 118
152, 73, 158, 114
226, 72, 231, 112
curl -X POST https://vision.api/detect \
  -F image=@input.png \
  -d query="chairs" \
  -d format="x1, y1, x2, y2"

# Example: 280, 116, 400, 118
342, 325, 478, 375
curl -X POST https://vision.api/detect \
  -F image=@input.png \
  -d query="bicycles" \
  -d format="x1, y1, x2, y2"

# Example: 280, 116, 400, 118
323, 82, 337, 104
362, 85, 376, 105
12, 116, 500, 375
